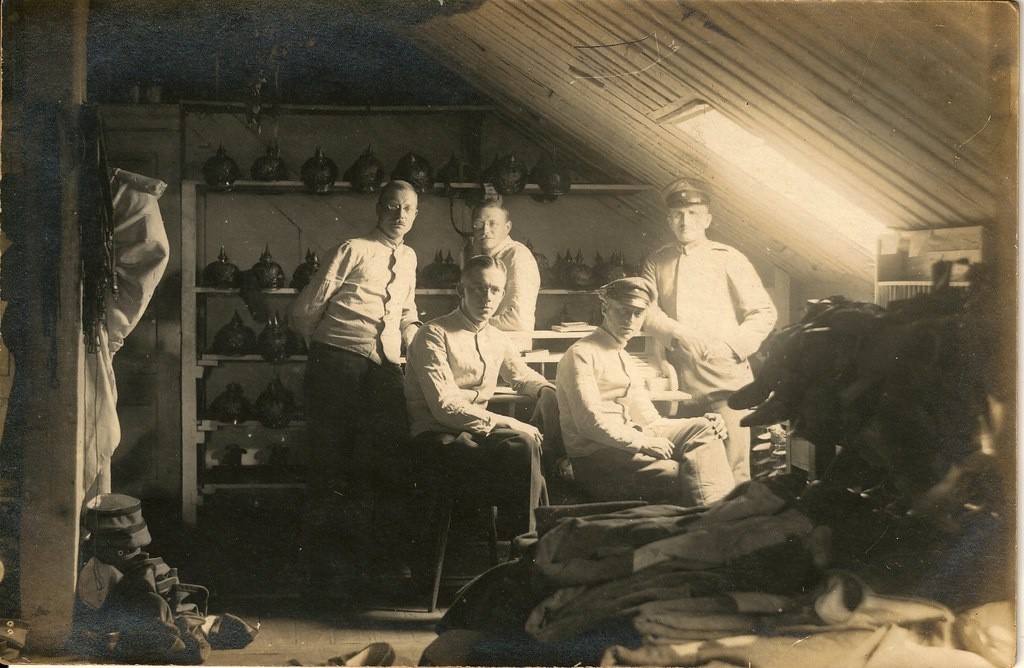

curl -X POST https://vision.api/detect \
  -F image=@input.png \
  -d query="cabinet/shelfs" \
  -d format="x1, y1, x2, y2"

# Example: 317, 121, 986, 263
181, 175, 658, 525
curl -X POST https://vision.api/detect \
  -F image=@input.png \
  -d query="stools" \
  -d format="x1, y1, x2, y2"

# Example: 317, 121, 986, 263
426, 503, 501, 613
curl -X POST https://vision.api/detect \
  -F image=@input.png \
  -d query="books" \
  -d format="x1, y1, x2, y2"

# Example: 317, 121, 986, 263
552, 322, 598, 332
522, 349, 549, 356
629, 353, 662, 390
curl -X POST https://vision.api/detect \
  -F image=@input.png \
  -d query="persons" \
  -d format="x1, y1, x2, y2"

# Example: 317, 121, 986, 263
556, 277, 737, 507
471, 201, 541, 330
290, 180, 426, 611
403, 255, 575, 562
640, 178, 777, 483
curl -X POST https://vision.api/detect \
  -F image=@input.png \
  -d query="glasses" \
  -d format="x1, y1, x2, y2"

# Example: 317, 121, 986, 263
470, 285, 505, 294
384, 202, 418, 213
473, 220, 504, 229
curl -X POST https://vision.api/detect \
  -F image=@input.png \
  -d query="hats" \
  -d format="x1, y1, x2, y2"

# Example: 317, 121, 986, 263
595, 277, 660, 309
660, 176, 715, 204
